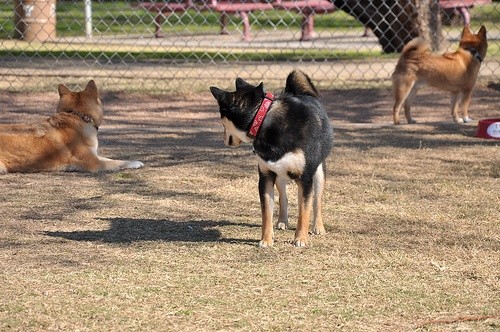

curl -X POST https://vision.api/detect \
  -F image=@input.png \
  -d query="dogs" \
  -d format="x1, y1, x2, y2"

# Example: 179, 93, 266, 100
0, 80, 144, 177
210, 70, 333, 247
391, 25, 487, 125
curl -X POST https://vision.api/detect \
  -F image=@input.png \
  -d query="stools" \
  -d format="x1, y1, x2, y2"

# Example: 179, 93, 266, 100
272, 0, 337, 42
439, 0, 491, 26
140, 1, 194, 36
195, 1, 273, 43
